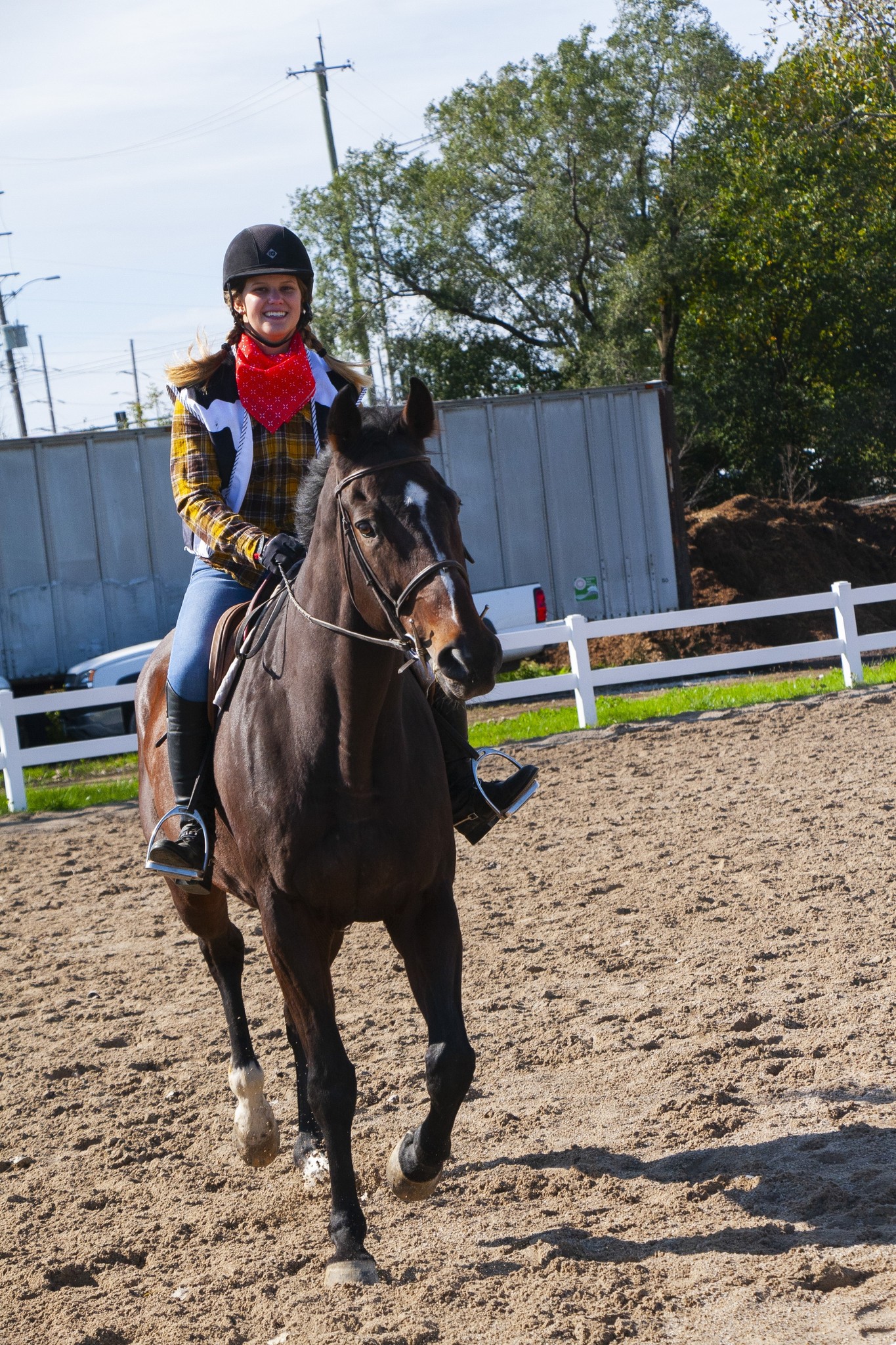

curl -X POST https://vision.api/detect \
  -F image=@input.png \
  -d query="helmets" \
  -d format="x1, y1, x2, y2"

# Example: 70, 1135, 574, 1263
222, 223, 314, 304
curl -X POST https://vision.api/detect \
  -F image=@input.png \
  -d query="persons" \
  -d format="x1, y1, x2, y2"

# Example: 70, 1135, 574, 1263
137, 220, 542, 879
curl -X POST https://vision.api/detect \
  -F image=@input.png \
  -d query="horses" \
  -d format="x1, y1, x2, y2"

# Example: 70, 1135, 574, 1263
132, 376, 502, 1285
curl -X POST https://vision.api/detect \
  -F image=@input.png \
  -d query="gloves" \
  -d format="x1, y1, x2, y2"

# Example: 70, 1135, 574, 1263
260, 532, 307, 578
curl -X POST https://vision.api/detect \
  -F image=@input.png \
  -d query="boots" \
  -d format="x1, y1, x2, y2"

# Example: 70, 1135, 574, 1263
147, 823, 215, 895
451, 765, 539, 845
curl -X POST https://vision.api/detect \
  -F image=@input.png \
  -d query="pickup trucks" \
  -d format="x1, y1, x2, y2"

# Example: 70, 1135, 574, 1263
65, 579, 551, 734
